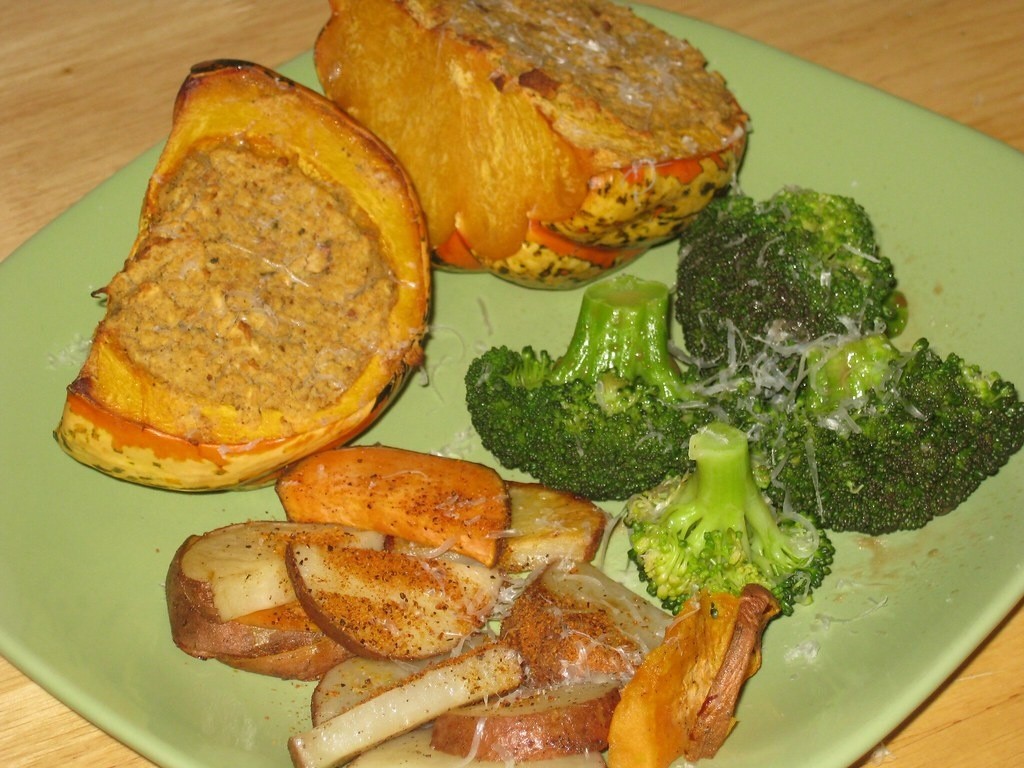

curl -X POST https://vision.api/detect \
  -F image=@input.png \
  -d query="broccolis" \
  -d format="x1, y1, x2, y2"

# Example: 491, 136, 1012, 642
463, 185, 1024, 617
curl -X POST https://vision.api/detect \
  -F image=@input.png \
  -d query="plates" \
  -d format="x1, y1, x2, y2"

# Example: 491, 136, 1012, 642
0, 0, 1024, 768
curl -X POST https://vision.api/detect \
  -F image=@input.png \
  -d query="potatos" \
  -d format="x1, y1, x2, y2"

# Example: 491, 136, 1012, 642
162, 519, 677, 768
